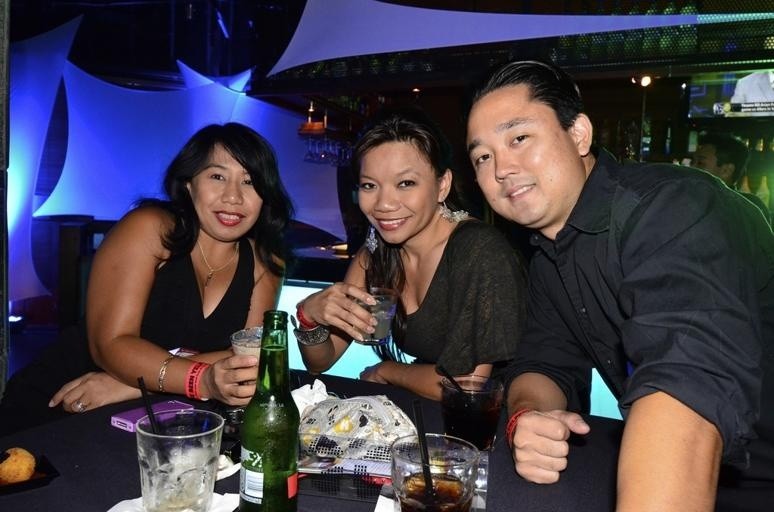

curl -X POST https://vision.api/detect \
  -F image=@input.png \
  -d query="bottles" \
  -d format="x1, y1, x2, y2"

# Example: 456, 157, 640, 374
327, 94, 376, 117
591, 116, 707, 169
240, 309, 300, 512
307, 100, 316, 123
323, 105, 330, 129
738, 132, 773, 212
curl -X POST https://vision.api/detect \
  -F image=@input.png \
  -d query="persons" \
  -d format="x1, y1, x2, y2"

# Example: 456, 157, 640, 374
2, 131, 309, 454
291, 117, 527, 403
689, 135, 750, 191
464, 58, 773, 512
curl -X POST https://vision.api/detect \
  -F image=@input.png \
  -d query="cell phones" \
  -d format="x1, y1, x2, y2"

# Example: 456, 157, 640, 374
110, 399, 194, 433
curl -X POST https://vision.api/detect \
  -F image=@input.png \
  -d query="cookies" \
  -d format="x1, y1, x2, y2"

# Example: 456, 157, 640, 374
0, 446, 36, 485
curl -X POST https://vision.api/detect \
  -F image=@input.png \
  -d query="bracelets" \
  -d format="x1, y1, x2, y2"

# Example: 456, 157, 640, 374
505, 408, 534, 447
295, 300, 319, 329
157, 352, 178, 394
290, 314, 331, 347
185, 359, 210, 403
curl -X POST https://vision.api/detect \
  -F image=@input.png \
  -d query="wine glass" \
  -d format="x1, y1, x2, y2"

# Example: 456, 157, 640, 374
298, 134, 354, 170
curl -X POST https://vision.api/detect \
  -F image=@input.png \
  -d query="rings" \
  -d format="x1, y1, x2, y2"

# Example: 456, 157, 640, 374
75, 400, 86, 411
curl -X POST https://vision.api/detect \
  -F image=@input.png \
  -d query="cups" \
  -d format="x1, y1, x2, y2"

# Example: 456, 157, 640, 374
348, 286, 398, 345
228, 326, 264, 385
388, 432, 481, 512
439, 374, 505, 453
135, 409, 226, 512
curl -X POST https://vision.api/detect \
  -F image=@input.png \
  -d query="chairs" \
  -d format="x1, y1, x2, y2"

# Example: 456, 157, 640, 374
58, 218, 120, 342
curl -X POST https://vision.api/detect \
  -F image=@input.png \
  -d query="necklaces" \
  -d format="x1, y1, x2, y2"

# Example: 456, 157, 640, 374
195, 235, 239, 286
